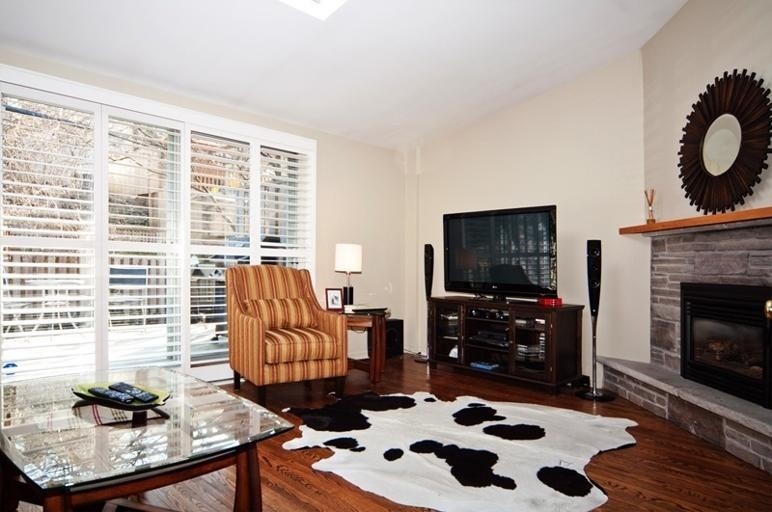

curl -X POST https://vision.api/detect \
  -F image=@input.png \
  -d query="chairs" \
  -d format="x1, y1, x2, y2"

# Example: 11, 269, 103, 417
226, 264, 348, 404
0, 267, 30, 334
107, 264, 148, 327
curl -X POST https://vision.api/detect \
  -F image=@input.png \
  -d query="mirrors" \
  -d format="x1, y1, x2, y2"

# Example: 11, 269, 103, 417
677, 68, 772, 216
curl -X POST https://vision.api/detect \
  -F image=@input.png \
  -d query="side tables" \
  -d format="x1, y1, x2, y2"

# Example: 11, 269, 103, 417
346, 314, 387, 375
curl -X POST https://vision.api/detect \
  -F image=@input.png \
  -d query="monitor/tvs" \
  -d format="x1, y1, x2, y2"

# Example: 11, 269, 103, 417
444, 205, 558, 303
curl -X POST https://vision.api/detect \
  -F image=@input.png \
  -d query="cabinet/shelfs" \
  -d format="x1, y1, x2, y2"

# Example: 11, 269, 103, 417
427, 296, 590, 396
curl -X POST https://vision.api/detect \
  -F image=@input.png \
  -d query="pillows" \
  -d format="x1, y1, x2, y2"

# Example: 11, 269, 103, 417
242, 297, 318, 330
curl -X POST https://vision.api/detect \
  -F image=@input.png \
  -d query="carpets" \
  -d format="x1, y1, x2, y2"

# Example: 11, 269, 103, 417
280, 389, 639, 512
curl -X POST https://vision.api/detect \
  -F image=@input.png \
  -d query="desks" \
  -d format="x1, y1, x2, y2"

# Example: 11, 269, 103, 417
25, 281, 81, 331
0, 366, 295, 512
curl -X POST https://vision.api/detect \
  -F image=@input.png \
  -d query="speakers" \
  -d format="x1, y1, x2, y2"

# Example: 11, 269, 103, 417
581, 240, 609, 403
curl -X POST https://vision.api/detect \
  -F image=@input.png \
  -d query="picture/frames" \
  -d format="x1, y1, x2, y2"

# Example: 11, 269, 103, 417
326, 288, 343, 311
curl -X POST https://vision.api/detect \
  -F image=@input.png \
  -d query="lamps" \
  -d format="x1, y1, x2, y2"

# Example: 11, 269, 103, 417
576, 240, 615, 400
336, 245, 362, 304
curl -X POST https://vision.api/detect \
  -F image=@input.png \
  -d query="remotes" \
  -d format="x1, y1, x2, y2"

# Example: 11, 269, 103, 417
88, 387, 134, 404
109, 382, 158, 403
